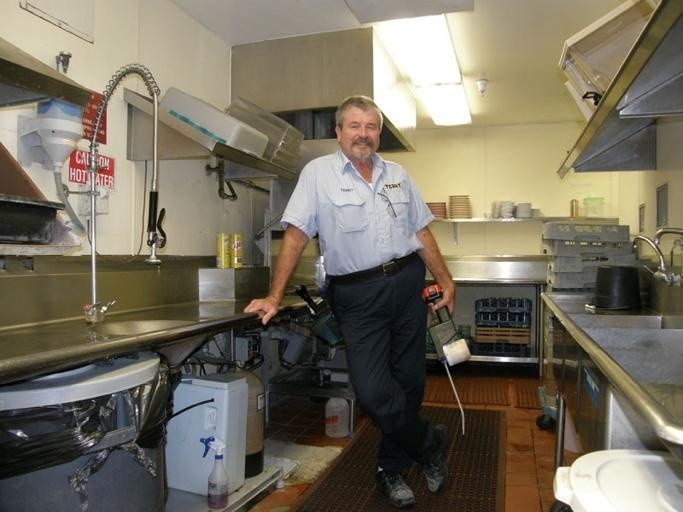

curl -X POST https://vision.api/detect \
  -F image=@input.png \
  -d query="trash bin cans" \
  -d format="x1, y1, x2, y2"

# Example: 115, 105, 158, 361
0, 351, 164, 512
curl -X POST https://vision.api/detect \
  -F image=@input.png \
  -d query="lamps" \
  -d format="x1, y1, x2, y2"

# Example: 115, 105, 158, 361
375, 14, 464, 83
477, 79, 489, 100
413, 84, 471, 127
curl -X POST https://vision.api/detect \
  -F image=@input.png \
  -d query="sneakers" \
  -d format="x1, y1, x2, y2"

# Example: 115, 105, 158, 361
374, 463, 418, 508
420, 425, 452, 494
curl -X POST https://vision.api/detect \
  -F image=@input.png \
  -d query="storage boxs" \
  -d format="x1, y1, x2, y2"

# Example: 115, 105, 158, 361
160, 88, 305, 170
559, 0, 657, 111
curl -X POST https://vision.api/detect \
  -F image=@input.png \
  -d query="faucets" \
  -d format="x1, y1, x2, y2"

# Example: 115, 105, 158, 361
652, 228, 679, 242
630, 236, 667, 274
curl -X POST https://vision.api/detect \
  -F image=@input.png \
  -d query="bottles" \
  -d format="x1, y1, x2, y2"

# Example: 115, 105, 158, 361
216, 231, 244, 269
572, 198, 579, 218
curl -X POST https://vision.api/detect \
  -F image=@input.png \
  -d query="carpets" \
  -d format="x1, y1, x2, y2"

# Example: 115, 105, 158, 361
422, 377, 511, 405
286, 405, 507, 512
513, 382, 542, 408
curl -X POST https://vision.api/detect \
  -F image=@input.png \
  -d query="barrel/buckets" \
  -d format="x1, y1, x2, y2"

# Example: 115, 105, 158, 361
585, 265, 640, 314
168, 372, 247, 494
326, 394, 351, 439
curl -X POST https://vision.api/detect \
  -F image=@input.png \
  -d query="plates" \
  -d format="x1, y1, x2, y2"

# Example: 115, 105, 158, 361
491, 200, 541, 219
426, 194, 473, 218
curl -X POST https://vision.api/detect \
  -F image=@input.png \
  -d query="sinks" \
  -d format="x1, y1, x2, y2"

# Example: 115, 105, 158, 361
569, 313, 681, 329
582, 326, 683, 385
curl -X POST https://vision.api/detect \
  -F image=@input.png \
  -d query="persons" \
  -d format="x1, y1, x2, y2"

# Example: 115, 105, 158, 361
242, 94, 455, 509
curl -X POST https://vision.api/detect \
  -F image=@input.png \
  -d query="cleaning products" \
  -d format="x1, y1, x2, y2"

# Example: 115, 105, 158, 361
202, 436, 227, 508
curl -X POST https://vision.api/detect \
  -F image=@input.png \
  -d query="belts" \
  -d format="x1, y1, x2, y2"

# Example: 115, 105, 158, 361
325, 251, 418, 287
